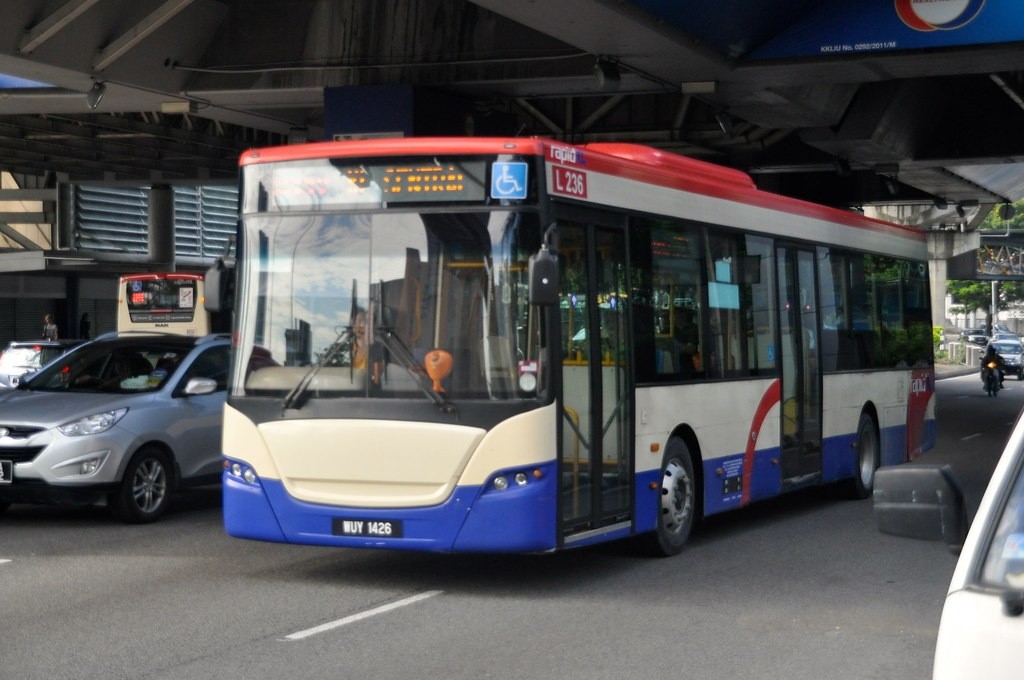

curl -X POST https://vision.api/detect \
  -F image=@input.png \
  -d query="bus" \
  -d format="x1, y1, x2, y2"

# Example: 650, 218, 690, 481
117, 273, 233, 339
223, 132, 937, 559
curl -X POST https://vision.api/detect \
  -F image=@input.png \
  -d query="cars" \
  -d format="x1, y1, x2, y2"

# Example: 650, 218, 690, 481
0, 341, 88, 390
978, 339, 1024, 381
958, 323, 1024, 347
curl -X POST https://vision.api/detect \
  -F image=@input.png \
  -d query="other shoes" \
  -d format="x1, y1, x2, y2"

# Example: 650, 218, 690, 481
999, 384, 1004, 388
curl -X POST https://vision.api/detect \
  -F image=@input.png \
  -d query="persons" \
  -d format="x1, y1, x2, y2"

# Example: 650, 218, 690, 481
42, 313, 58, 342
80, 313, 90, 340
597, 298, 703, 372
980, 343, 1006, 390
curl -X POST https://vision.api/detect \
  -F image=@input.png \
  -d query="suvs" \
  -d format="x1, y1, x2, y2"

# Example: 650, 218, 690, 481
0, 328, 281, 525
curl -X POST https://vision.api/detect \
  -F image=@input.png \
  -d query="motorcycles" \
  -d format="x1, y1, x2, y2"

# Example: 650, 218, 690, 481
978, 357, 1003, 398
